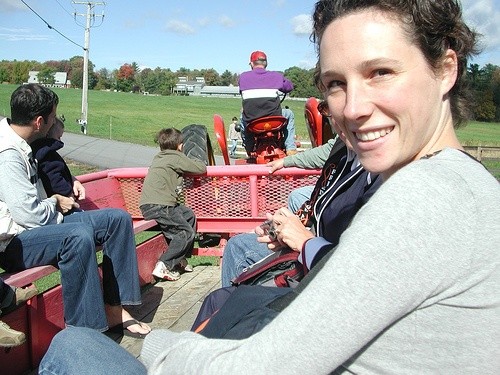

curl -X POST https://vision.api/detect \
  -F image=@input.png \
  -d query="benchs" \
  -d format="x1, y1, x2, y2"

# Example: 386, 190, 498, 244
0, 164, 323, 375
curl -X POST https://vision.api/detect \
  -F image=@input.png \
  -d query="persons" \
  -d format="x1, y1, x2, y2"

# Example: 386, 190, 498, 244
0, 83, 153, 347
238, 50, 297, 155
39, 0, 500, 374
229, 117, 241, 156
189, 100, 384, 341
139, 128, 206, 282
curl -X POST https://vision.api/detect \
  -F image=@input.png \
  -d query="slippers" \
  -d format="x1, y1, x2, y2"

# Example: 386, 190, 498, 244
110, 319, 150, 340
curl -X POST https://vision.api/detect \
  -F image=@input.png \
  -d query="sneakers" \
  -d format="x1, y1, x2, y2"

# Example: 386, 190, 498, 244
1, 319, 27, 347
286, 149, 297, 155
152, 260, 181, 280
183, 264, 194, 271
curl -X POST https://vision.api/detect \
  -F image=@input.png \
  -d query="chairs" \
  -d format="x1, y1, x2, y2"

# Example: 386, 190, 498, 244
245, 116, 290, 142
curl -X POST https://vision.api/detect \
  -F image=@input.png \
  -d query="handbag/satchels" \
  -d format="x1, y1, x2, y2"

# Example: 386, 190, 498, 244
233, 245, 305, 287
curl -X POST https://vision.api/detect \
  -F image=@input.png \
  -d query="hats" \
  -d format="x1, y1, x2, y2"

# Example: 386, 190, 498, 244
249, 51, 267, 63
